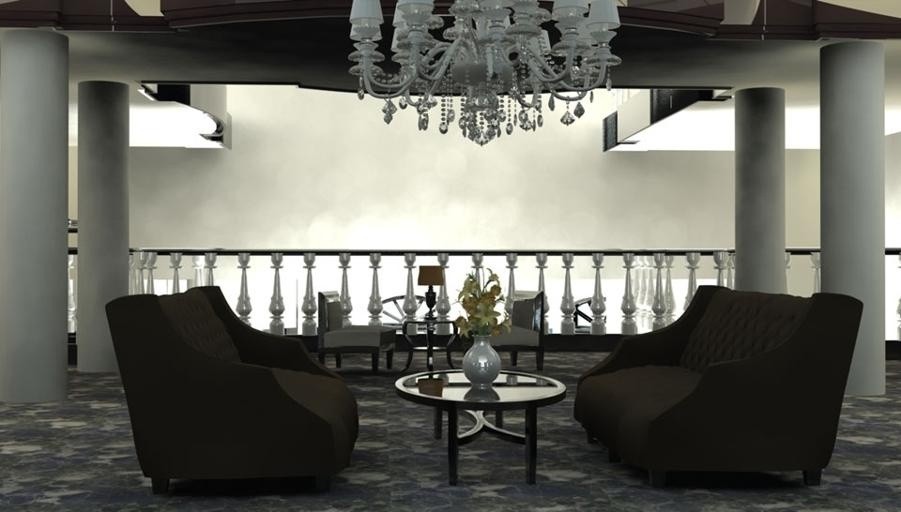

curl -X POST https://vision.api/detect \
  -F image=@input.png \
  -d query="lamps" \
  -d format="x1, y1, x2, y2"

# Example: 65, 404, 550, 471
348, 0, 622, 145
419, 266, 444, 320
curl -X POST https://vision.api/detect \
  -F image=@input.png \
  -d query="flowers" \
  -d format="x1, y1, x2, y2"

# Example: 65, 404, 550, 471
455, 268, 512, 339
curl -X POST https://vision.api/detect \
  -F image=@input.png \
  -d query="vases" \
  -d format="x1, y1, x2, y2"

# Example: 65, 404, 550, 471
462, 335, 502, 389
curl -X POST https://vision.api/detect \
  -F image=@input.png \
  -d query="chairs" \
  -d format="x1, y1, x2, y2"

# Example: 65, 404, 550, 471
492, 292, 545, 371
319, 291, 396, 373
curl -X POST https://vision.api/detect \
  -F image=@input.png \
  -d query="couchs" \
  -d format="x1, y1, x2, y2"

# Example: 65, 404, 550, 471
574, 285, 864, 486
106, 286, 358, 493
